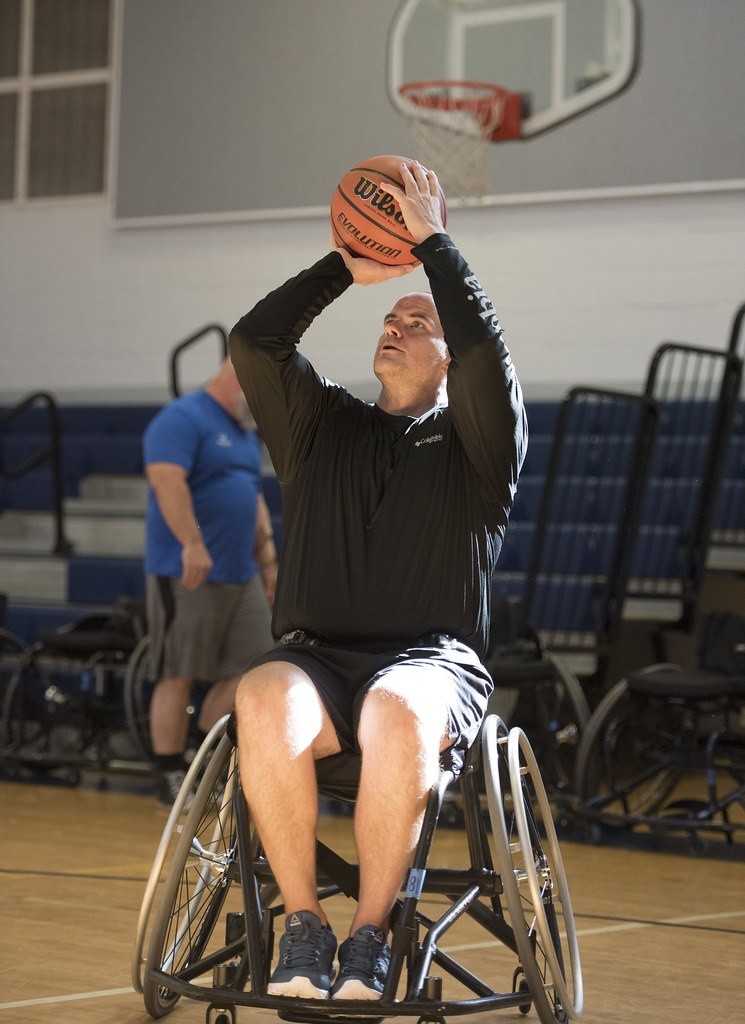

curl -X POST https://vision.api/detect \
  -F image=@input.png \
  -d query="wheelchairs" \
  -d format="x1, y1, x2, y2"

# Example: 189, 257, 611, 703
127, 706, 589, 1024
1, 587, 745, 855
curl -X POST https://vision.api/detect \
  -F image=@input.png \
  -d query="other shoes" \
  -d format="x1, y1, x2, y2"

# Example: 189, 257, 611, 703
194, 777, 226, 805
151, 770, 192, 811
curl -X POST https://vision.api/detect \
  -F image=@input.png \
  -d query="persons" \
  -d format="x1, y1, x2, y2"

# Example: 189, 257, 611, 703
142, 357, 276, 808
224, 188, 530, 1000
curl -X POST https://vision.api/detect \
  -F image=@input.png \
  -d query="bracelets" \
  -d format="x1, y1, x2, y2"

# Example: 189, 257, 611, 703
259, 555, 279, 573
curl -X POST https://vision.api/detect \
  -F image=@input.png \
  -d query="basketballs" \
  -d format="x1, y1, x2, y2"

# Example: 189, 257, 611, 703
331, 153, 447, 268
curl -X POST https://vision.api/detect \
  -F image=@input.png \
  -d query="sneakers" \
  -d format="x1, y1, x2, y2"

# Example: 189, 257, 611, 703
267, 911, 337, 1000
329, 924, 400, 1018
